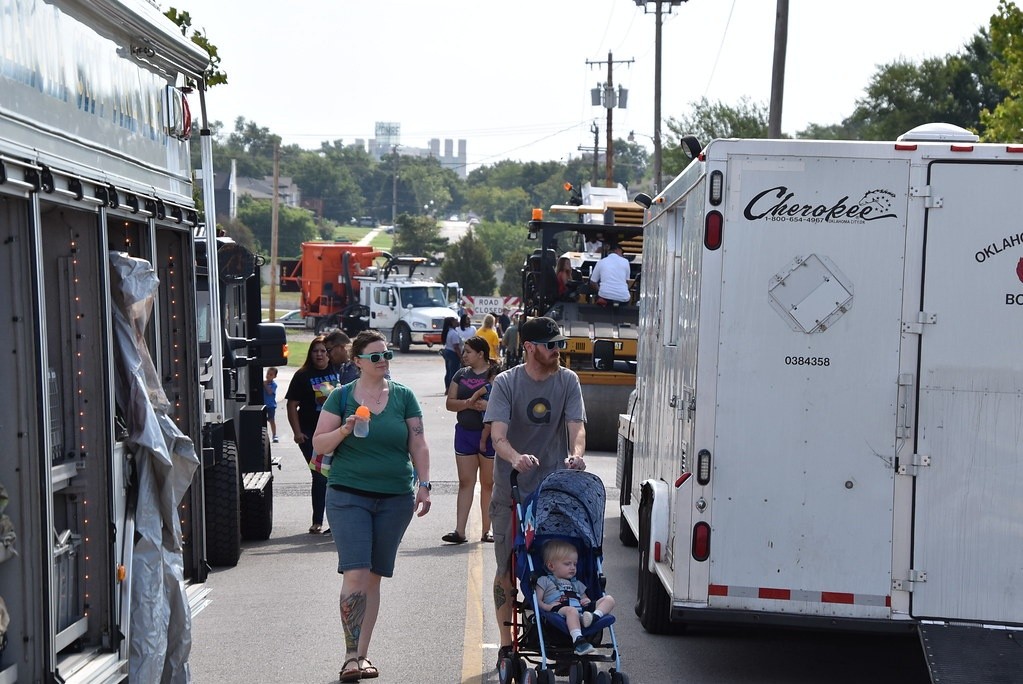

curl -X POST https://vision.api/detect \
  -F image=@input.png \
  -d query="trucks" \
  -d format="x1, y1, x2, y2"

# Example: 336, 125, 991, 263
300, 243, 461, 353
615, 122, 1022, 640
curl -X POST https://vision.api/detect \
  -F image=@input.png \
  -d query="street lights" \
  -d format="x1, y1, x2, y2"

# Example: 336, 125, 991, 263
627, 132, 663, 197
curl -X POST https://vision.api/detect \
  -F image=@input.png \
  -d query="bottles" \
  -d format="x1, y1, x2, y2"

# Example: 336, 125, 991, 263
353, 398, 371, 438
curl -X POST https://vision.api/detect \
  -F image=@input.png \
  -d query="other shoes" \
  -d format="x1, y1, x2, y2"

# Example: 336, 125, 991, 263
310, 524, 322, 533
325, 528, 331, 536
496, 645, 513, 671
273, 436, 279, 443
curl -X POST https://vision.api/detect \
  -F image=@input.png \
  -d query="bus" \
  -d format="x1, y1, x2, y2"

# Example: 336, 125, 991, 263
0, 0, 211, 684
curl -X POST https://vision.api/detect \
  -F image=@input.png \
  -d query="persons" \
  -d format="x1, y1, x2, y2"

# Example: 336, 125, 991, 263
312, 330, 431, 681
438, 301, 519, 542
556, 257, 580, 304
483, 317, 587, 670
586, 231, 602, 253
263, 367, 279, 443
284, 330, 361, 536
590, 243, 631, 305
536, 539, 615, 656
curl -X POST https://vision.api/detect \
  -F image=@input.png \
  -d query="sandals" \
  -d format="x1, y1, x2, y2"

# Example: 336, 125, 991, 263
339, 657, 379, 682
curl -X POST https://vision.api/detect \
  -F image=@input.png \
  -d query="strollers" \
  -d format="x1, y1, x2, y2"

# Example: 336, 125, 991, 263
499, 456, 631, 684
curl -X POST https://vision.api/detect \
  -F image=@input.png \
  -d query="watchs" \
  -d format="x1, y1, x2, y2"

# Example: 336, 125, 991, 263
419, 482, 431, 491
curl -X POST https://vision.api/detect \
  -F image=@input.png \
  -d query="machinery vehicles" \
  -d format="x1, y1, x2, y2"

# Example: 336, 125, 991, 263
506, 181, 644, 450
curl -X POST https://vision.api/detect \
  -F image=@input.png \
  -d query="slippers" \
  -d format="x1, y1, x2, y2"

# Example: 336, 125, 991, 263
480, 531, 494, 541
442, 531, 468, 543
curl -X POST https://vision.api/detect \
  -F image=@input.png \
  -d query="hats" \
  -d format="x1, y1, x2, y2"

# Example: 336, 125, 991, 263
520, 318, 571, 344
503, 307, 511, 313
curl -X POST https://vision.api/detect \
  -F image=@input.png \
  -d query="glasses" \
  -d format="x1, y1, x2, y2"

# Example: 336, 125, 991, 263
531, 341, 565, 350
356, 352, 393, 364
326, 343, 340, 353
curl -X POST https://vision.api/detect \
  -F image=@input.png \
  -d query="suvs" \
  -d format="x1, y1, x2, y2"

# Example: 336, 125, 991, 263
192, 222, 290, 564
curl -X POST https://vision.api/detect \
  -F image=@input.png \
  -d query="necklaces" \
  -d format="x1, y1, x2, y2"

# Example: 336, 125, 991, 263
361, 380, 384, 404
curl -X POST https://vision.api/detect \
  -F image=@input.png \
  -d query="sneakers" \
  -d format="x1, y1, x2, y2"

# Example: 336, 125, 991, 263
574, 635, 595, 655
583, 611, 600, 628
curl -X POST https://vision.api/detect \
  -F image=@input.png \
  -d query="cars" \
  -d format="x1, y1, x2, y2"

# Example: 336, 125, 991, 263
466, 215, 474, 222
261, 310, 306, 322
358, 217, 377, 227
470, 219, 479, 225
386, 224, 400, 234
450, 216, 458, 221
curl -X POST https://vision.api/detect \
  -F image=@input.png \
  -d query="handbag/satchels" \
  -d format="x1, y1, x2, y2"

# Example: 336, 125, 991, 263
308, 448, 334, 477
